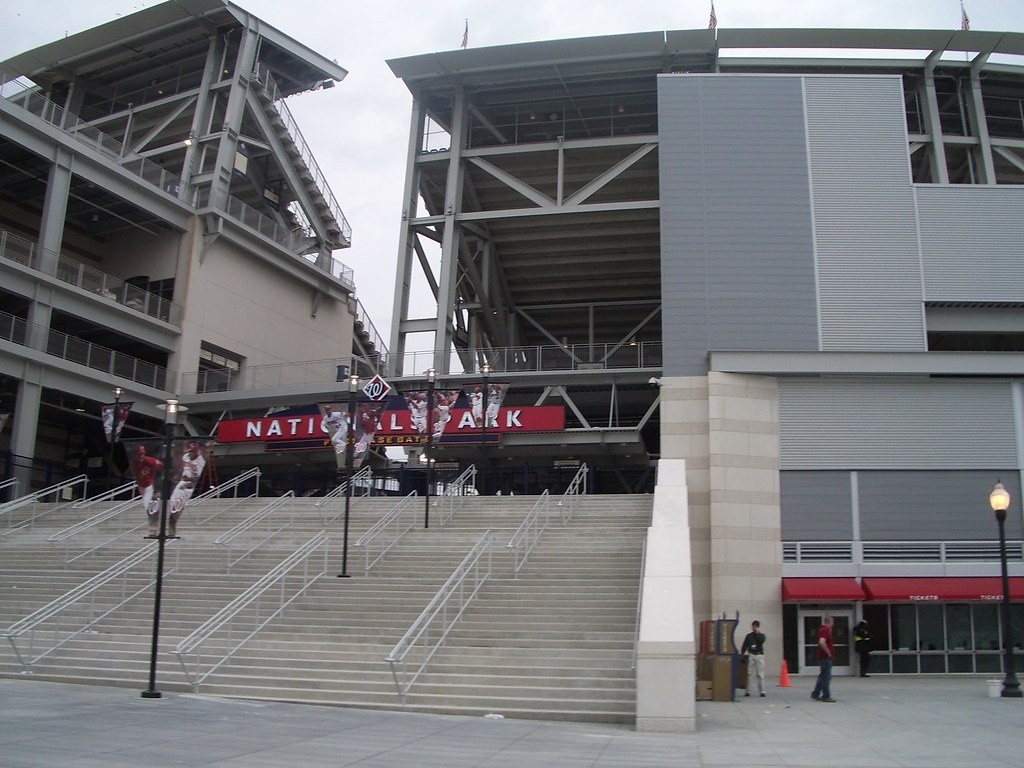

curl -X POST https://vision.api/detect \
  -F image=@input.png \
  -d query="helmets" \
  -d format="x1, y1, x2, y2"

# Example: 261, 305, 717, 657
416, 394, 426, 399
367, 409, 376, 415
135, 444, 145, 456
496, 385, 501, 390
438, 394, 445, 403
188, 443, 199, 452
121, 406, 126, 413
324, 405, 332, 412
474, 387, 480, 393
103, 406, 109, 412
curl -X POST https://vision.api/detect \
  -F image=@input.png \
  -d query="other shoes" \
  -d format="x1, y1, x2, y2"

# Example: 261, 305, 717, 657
821, 696, 836, 703
811, 692, 822, 700
745, 692, 750, 696
860, 673, 869, 677
761, 692, 766, 696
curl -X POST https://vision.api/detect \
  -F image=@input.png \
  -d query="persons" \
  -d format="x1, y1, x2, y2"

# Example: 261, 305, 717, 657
853, 620, 872, 677
320, 385, 505, 469
102, 402, 207, 539
739, 620, 766, 697
811, 616, 836, 702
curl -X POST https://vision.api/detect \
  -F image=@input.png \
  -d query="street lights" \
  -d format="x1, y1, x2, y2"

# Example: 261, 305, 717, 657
480, 361, 495, 498
776, 659, 792, 690
988, 480, 1024, 698
333, 373, 359, 580
139, 386, 187, 698
421, 368, 441, 530
107, 388, 125, 493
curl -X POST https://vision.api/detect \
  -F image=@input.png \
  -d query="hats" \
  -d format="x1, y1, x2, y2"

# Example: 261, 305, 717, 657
752, 620, 760, 626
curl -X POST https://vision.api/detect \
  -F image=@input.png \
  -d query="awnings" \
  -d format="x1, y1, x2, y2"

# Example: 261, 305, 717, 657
781, 578, 866, 602
862, 575, 1024, 603
